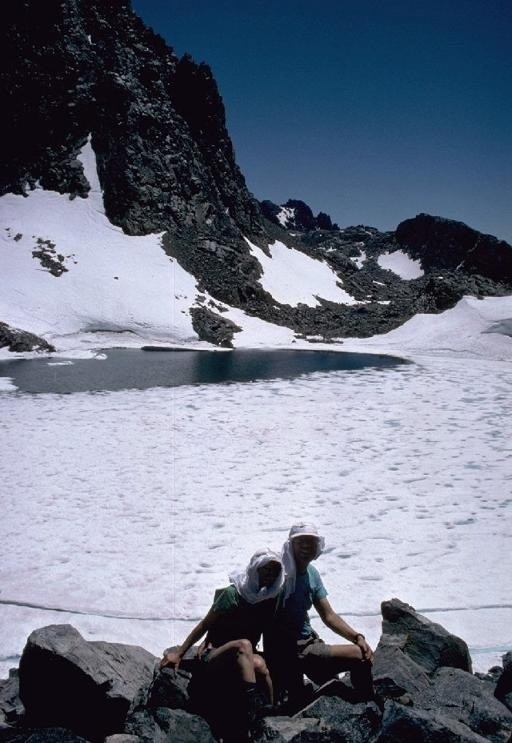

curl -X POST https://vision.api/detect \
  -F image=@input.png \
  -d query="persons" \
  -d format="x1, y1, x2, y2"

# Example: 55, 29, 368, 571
161, 545, 285, 707
263, 521, 375, 699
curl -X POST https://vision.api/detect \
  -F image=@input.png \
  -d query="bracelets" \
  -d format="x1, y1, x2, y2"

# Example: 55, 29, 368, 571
352, 633, 365, 644
